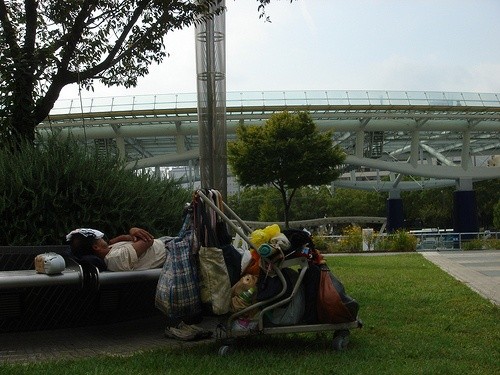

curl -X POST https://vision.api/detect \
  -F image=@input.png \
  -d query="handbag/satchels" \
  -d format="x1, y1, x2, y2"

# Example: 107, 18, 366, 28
153, 239, 198, 317
198, 246, 230, 302
265, 262, 359, 326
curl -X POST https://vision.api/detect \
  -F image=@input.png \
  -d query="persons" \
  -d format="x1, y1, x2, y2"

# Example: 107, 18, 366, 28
66, 227, 174, 272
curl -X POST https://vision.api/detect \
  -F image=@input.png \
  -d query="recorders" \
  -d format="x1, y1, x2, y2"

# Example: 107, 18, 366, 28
35, 252, 65, 275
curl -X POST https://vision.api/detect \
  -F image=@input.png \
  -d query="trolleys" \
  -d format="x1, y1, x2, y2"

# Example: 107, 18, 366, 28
197, 186, 364, 355
